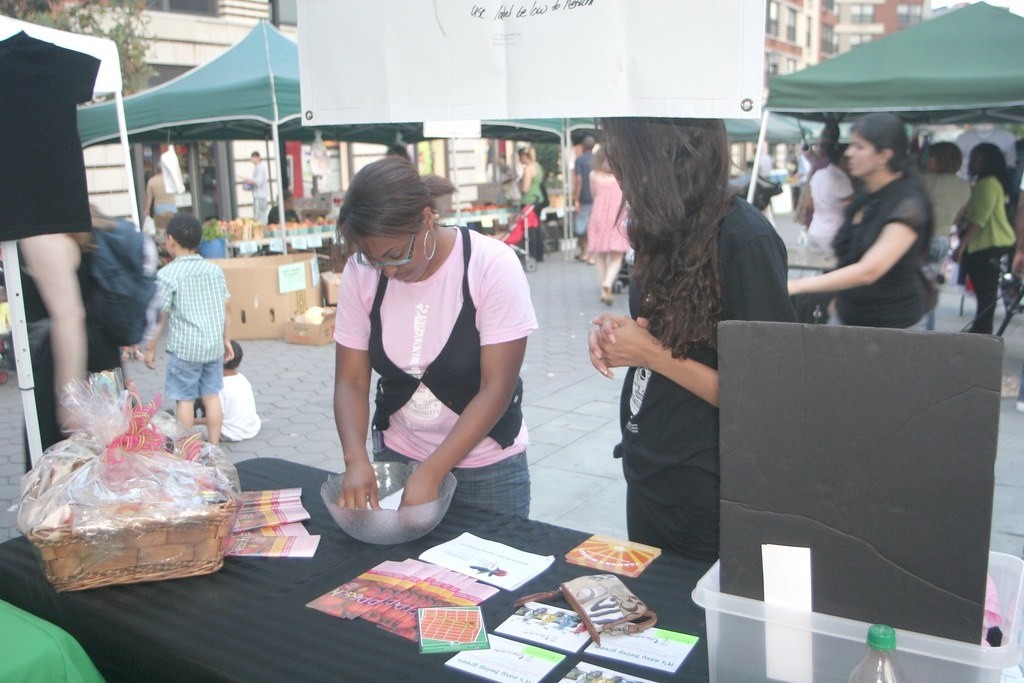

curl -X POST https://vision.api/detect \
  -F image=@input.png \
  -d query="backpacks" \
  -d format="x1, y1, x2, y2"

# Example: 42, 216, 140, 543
85, 217, 154, 347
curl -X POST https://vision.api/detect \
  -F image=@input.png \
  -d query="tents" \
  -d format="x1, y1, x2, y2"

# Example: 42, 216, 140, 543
0, 12, 933, 257
748, 0, 1024, 205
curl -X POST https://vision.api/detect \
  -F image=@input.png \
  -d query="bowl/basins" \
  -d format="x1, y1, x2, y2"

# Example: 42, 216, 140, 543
320, 461, 457, 545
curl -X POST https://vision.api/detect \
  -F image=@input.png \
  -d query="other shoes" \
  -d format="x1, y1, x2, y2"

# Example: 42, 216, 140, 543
615, 281, 623, 294
1016, 401, 1024, 412
600, 282, 613, 306
579, 253, 596, 264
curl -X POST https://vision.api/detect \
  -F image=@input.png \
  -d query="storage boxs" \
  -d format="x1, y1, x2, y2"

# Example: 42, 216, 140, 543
228, 207, 521, 243
204, 252, 321, 340
558, 237, 579, 253
321, 272, 342, 306
691, 550, 1024, 683
549, 196, 562, 207
282, 306, 336, 347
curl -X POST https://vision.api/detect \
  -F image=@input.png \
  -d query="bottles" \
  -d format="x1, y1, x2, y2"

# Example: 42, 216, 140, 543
849, 624, 910, 683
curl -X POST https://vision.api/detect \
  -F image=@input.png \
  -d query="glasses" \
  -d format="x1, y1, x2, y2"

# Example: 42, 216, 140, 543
357, 234, 416, 266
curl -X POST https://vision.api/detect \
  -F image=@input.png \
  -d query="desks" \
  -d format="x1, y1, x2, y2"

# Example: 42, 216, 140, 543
770, 211, 839, 271
541, 205, 576, 223
228, 212, 517, 272
0, 457, 715, 683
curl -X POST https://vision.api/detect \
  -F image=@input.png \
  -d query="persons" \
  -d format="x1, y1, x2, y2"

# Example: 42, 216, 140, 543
120, 344, 145, 363
753, 148, 773, 180
574, 135, 631, 307
788, 114, 935, 333
18, 204, 128, 476
266, 190, 298, 223
517, 145, 544, 262
144, 160, 178, 233
190, 340, 262, 442
744, 137, 1024, 413
243, 152, 270, 223
145, 211, 234, 448
333, 156, 540, 519
386, 146, 413, 166
589, 118, 800, 563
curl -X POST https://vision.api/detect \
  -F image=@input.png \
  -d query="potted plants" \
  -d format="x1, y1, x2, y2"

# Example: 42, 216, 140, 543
197, 218, 235, 259
